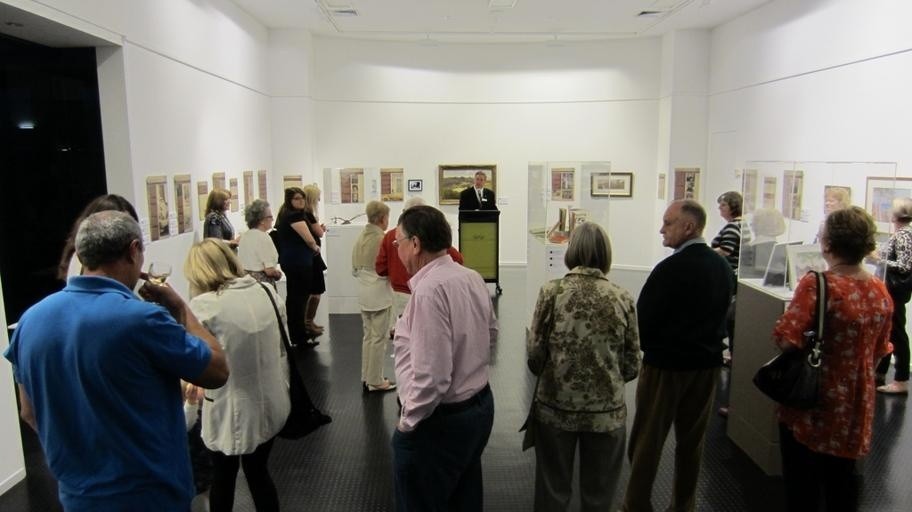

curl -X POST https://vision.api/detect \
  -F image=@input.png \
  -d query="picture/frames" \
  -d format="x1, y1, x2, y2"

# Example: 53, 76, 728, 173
408, 180, 422, 191
865, 176, 912, 235
787, 244, 827, 290
591, 172, 633, 196
439, 165, 497, 205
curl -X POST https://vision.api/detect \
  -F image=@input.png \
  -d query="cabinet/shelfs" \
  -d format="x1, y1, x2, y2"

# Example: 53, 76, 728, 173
727, 278, 800, 476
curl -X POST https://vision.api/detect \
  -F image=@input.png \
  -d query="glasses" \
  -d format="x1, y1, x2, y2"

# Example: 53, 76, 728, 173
393, 237, 406, 247
266, 216, 273, 220
293, 197, 305, 200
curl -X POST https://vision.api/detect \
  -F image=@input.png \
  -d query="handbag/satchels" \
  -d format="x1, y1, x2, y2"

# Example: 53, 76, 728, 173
279, 344, 333, 440
887, 232, 912, 301
520, 402, 535, 450
754, 269, 827, 406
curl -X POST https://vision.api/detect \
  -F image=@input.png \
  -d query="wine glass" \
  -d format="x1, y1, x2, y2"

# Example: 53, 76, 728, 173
148, 261, 170, 286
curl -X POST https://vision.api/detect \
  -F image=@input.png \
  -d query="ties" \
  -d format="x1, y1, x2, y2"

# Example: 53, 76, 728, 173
478, 190, 482, 201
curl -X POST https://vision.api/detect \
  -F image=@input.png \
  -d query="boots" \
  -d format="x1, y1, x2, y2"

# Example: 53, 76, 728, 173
306, 297, 323, 335
312, 322, 323, 332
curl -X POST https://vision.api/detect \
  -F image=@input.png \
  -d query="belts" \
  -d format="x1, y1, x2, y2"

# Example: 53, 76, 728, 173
441, 383, 489, 414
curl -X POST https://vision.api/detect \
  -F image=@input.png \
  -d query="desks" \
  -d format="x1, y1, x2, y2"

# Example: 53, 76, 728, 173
325, 225, 365, 314
526, 231, 569, 318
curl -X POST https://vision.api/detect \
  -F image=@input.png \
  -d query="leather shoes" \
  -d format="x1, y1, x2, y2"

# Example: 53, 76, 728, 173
369, 383, 396, 391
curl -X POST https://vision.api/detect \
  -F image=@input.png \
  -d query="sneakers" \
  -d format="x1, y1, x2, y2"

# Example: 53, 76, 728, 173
877, 380, 909, 394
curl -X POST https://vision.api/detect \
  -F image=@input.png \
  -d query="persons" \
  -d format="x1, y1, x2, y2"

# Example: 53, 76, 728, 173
457, 171, 496, 211
525, 222, 641, 512
618, 198, 734, 511
711, 191, 758, 356
352, 197, 499, 512
770, 207, 894, 512
864, 197, 912, 394
2, 173, 325, 512
